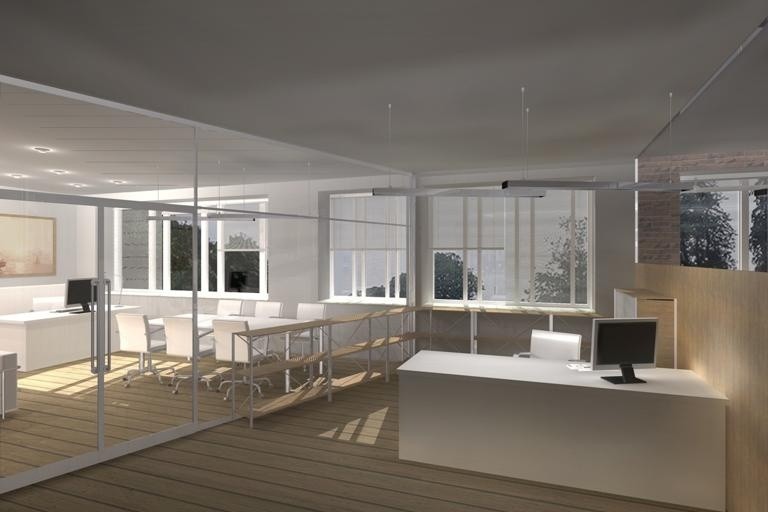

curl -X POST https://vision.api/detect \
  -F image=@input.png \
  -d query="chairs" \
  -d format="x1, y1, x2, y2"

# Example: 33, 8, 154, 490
116, 299, 325, 401
31, 296, 65, 311
515, 328, 583, 362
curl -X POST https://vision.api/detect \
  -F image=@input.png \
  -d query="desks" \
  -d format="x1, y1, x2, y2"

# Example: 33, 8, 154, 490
0, 304, 142, 373
396, 348, 730, 511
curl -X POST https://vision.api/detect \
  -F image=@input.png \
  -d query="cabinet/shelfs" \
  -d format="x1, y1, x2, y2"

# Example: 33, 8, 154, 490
230, 305, 432, 428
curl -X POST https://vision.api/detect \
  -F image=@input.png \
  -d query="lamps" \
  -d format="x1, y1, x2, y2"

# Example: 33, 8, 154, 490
207, 161, 312, 220
501, 86, 693, 193
371, 86, 545, 199
144, 164, 213, 220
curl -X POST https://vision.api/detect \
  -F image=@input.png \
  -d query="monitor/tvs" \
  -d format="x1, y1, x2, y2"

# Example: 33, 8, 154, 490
65, 276, 96, 315
588, 314, 660, 384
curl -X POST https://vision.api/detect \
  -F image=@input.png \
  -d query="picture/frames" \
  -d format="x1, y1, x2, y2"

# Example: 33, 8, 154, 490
0, 212, 56, 279
